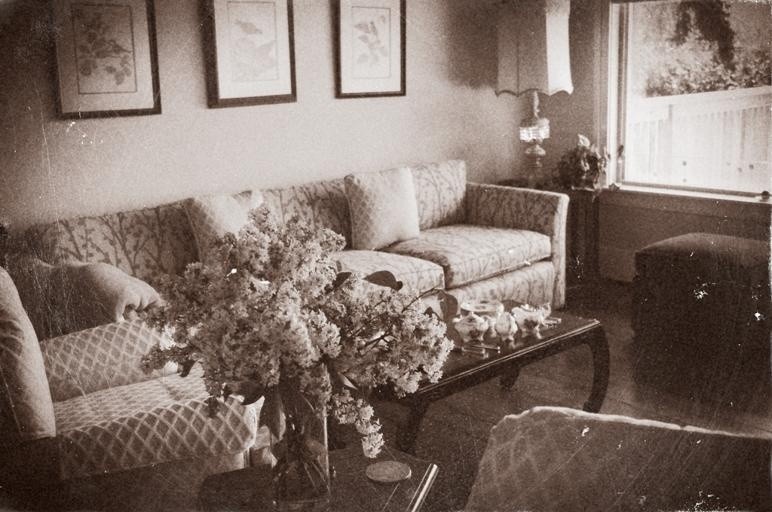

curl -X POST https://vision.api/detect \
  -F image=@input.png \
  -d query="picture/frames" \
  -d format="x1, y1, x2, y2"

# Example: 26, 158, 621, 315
42, 0, 162, 120
333, 0, 407, 99
199, 0, 298, 109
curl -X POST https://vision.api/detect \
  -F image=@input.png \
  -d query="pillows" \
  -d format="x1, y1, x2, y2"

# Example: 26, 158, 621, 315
183, 187, 265, 262
344, 164, 421, 249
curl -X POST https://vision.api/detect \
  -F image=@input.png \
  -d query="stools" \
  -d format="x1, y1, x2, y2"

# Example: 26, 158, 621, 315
629, 230, 772, 362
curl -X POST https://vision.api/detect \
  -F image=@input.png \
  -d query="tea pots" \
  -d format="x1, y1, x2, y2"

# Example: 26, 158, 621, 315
456, 312, 518, 344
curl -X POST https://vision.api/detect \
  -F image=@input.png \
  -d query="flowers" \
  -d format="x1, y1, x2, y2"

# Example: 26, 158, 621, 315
121, 202, 452, 461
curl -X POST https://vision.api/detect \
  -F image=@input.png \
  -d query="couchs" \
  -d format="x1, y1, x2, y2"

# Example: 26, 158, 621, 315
4, 158, 569, 448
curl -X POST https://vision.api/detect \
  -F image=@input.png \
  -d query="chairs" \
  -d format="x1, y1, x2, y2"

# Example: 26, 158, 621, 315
0, 270, 275, 510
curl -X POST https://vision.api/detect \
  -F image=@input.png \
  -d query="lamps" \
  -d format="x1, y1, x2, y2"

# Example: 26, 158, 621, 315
493, 0, 576, 191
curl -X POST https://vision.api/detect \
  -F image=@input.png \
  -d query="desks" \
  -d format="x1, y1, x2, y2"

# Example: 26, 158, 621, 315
499, 179, 601, 283
197, 440, 441, 512
